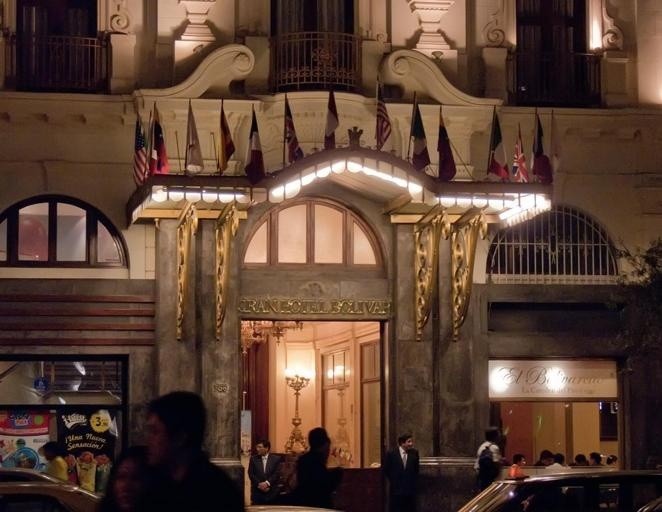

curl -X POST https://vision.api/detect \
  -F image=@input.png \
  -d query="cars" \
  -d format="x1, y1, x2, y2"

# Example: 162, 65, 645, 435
1, 478, 101, 512
0, 466, 62, 483
635, 495, 662, 512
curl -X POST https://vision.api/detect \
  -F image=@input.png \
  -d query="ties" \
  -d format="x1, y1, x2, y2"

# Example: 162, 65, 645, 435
403, 451, 407, 469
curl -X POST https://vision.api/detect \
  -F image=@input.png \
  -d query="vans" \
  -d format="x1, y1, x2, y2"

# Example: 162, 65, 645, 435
454, 468, 662, 512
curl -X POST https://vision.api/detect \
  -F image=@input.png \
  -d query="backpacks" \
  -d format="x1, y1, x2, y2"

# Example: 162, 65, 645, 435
477, 442, 500, 483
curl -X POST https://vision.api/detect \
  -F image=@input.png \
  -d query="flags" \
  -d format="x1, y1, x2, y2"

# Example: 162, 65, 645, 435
216, 107, 236, 172
511, 123, 530, 184
373, 79, 392, 152
408, 95, 432, 170
324, 86, 341, 150
529, 113, 553, 186
145, 103, 169, 175
185, 103, 204, 175
131, 113, 149, 188
486, 108, 511, 182
284, 96, 304, 163
243, 110, 267, 186
435, 110, 458, 181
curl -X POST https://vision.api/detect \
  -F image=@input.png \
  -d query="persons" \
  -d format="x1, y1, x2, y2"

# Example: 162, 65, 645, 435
386, 431, 421, 512
508, 448, 619, 480
128, 390, 246, 512
246, 439, 283, 505
92, 444, 148, 512
41, 441, 68, 482
472, 425, 505, 493
282, 426, 343, 509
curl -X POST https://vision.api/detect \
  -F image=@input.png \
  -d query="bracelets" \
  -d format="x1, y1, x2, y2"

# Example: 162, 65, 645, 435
265, 482, 269, 487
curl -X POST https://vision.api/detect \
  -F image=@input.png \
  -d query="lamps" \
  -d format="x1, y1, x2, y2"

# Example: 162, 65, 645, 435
434, 193, 515, 210
249, 318, 304, 349
265, 156, 426, 201
152, 186, 246, 206
